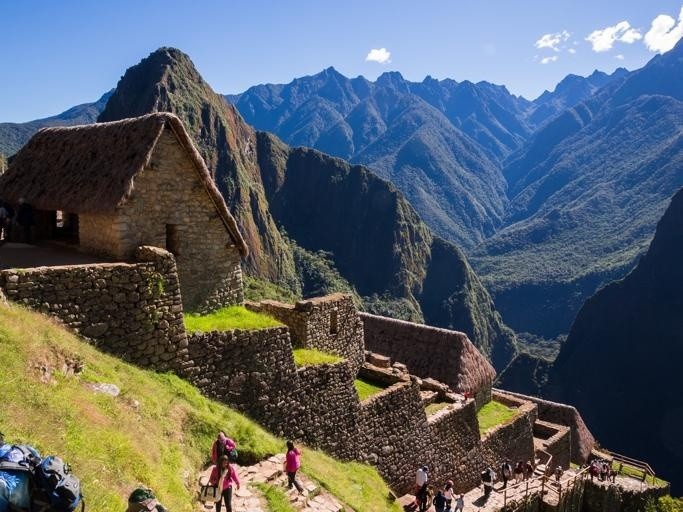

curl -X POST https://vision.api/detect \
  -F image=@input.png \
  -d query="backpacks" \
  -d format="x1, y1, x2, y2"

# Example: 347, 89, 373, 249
0, 444, 84, 512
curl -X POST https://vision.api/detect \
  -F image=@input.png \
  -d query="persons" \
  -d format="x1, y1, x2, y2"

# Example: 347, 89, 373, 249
124, 489, 167, 512
555, 466, 563, 481
416, 458, 534, 512
576, 456, 617, 483
283, 441, 304, 495
209, 455, 240, 512
211, 432, 239, 464
0, 199, 7, 240
0, 431, 85, 512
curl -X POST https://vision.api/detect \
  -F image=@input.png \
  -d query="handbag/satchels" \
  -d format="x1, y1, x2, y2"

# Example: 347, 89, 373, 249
201, 486, 221, 502
229, 450, 237, 460
